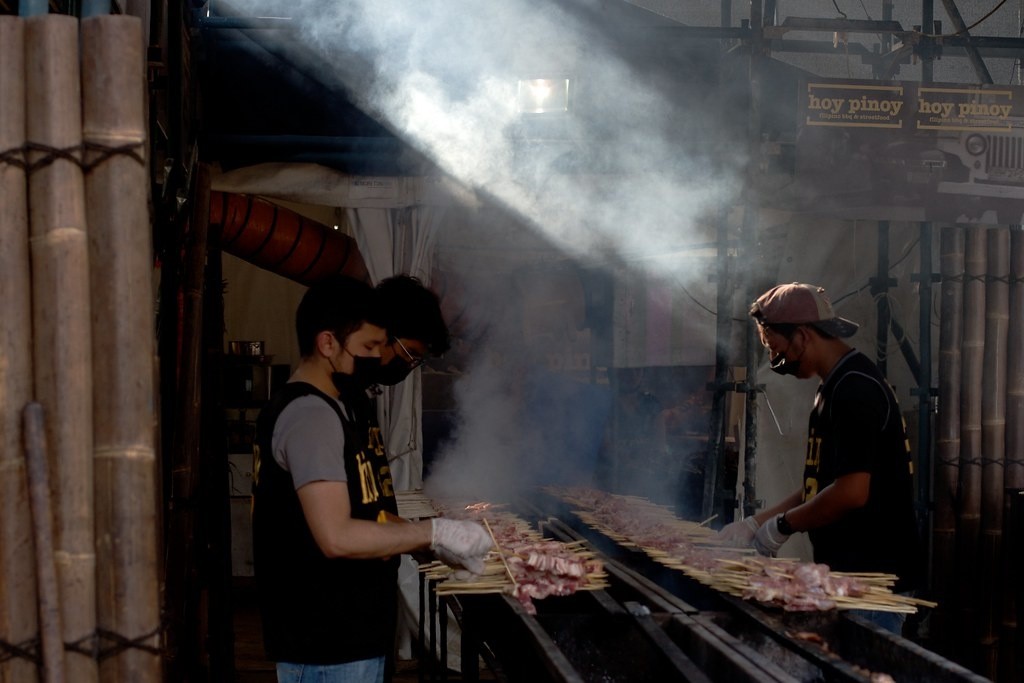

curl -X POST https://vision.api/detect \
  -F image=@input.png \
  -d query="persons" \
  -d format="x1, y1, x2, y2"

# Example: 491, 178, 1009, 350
720, 281, 920, 636
260, 273, 499, 683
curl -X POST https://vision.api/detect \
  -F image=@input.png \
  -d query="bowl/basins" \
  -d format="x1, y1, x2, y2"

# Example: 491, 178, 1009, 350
228, 340, 264, 356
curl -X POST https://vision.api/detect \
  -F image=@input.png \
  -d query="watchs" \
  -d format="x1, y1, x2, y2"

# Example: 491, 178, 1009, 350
776, 512, 794, 535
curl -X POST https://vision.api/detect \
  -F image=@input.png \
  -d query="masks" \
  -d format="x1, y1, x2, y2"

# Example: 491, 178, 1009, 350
329, 338, 382, 394
770, 345, 806, 379
377, 345, 412, 386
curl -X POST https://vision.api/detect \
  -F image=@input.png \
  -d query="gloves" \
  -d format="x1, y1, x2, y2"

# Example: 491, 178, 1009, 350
431, 516, 492, 575
755, 514, 791, 558
708, 516, 759, 549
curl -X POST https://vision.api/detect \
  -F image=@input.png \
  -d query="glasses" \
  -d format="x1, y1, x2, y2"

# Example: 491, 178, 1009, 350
394, 337, 427, 368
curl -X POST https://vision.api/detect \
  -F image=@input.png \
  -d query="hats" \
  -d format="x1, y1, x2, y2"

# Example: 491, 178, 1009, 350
757, 282, 859, 338
